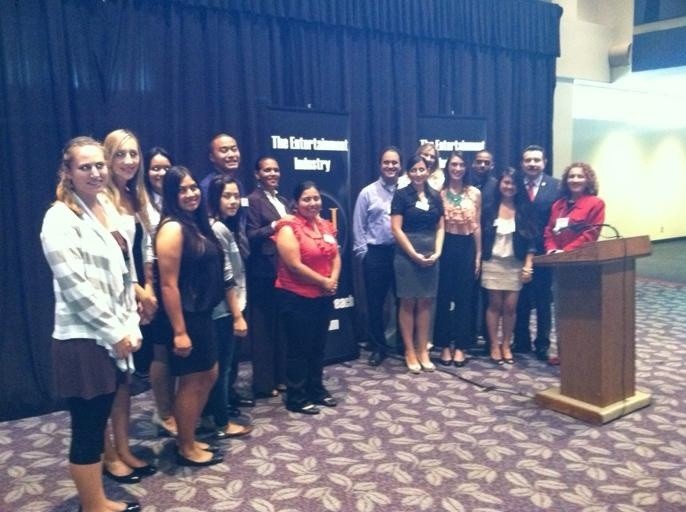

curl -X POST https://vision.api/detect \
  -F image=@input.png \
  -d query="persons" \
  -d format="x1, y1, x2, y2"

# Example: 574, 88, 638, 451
208, 175, 252, 438
151, 164, 224, 465
242, 154, 295, 397
199, 131, 256, 417
39, 134, 143, 512
143, 146, 178, 438
272, 180, 342, 415
96, 127, 157, 482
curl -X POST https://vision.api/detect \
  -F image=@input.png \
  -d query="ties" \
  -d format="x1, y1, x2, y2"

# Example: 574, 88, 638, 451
527, 183, 535, 200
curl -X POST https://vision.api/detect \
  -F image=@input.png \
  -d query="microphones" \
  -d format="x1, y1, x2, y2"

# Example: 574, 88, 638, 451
551, 222, 619, 239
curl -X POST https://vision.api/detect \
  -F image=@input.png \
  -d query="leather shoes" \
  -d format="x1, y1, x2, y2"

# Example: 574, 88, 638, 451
219, 383, 287, 438
367, 339, 560, 373
102, 461, 141, 484
297, 402, 320, 414
79, 498, 142, 512
318, 394, 337, 406
127, 458, 157, 476
176, 445, 224, 466
174, 439, 221, 455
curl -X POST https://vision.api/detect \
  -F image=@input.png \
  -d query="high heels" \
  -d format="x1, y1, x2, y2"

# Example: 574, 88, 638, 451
151, 408, 178, 439
